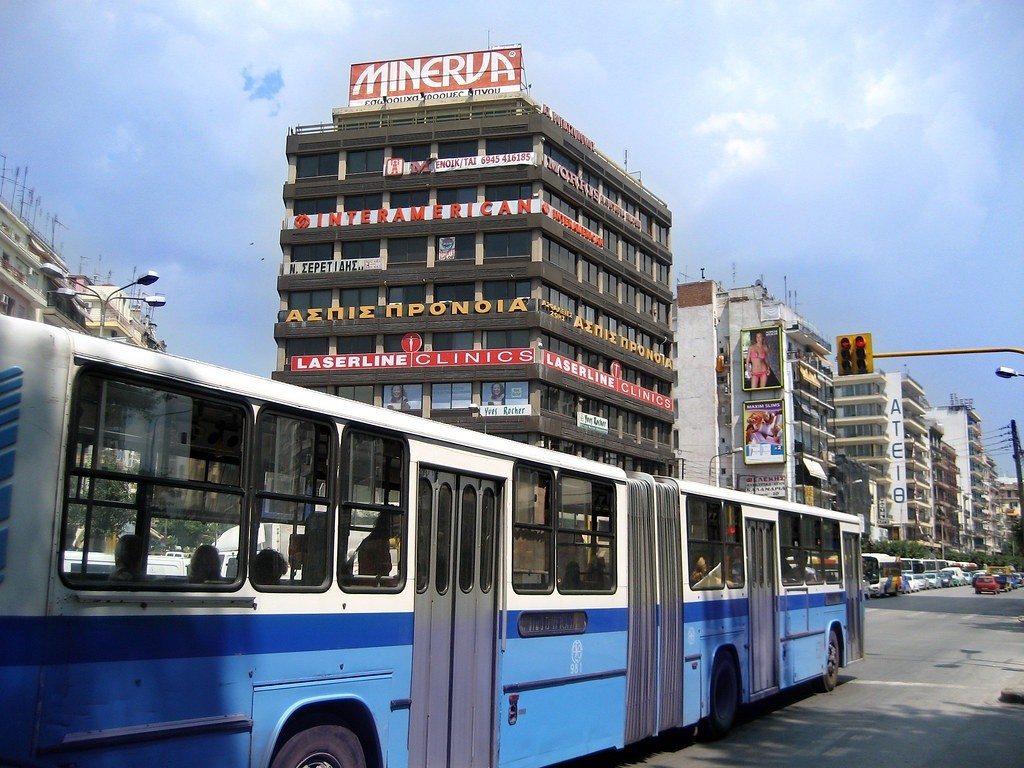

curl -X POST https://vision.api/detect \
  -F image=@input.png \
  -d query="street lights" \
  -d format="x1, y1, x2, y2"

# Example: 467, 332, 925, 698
39, 258, 168, 584
468, 402, 487, 434
708, 447, 744, 485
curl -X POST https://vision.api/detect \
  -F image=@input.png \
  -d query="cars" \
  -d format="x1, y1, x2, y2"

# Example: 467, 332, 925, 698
975, 576, 1001, 595
902, 566, 1024, 601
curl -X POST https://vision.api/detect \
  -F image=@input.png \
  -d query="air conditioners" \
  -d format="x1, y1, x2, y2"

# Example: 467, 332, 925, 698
0, 294, 9, 305
28, 267, 35, 277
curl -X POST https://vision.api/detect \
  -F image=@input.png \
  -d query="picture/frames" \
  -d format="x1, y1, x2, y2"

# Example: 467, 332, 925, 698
740, 326, 784, 391
742, 399, 787, 465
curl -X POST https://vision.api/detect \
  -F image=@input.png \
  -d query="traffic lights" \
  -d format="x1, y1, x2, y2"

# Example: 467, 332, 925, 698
836, 334, 854, 377
853, 331, 874, 373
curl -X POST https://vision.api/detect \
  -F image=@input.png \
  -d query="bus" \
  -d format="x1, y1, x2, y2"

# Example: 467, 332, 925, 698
1, 315, 868, 768
923, 560, 978, 572
900, 558, 926, 574
859, 552, 902, 599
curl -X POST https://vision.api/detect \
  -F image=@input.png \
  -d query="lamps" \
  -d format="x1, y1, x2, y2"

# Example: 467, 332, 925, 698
383, 96, 386, 102
469, 88, 473, 96
420, 92, 426, 99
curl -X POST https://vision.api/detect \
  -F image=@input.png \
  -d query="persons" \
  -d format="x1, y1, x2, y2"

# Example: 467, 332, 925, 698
110, 504, 817, 589
746, 331, 771, 388
489, 383, 504, 402
390, 385, 410, 409
